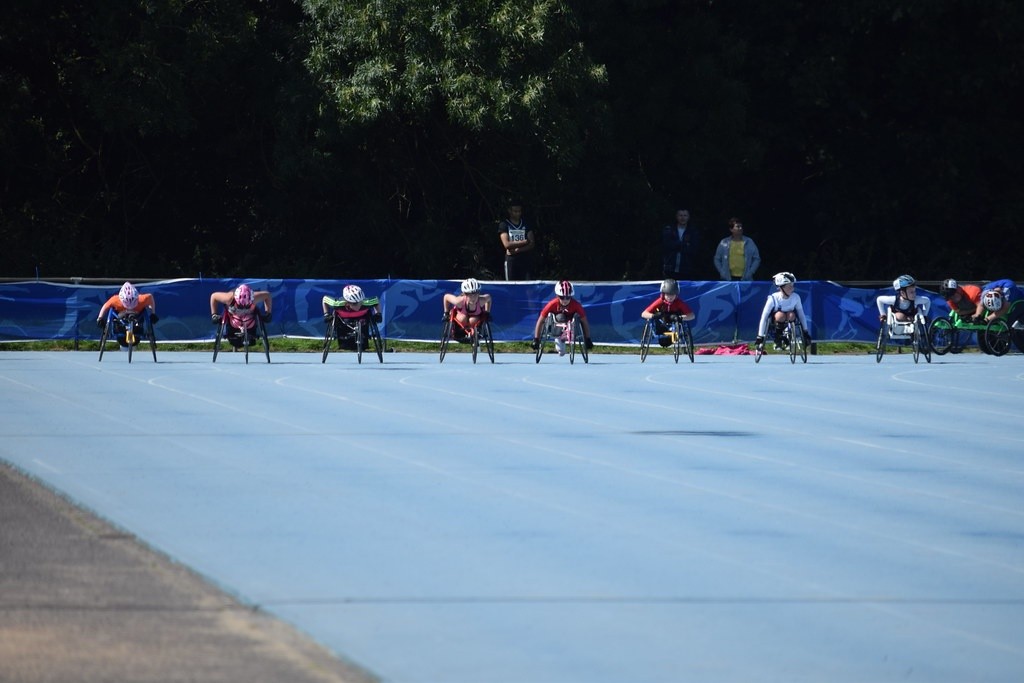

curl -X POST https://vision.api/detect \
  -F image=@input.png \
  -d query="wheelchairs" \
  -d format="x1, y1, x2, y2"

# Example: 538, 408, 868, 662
440, 309, 494, 364
322, 309, 383, 364
213, 310, 271, 364
929, 300, 1024, 356
755, 313, 807, 364
98, 306, 157, 363
876, 312, 931, 363
536, 312, 589, 363
640, 312, 694, 363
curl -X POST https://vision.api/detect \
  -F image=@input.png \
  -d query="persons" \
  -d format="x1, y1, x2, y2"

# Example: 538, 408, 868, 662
754, 272, 812, 350
939, 278, 1024, 322
876, 275, 930, 344
97, 280, 156, 347
660, 208, 702, 279
210, 284, 271, 348
641, 279, 695, 347
498, 201, 536, 280
441, 278, 492, 344
322, 284, 381, 351
713, 218, 762, 281
532, 280, 593, 350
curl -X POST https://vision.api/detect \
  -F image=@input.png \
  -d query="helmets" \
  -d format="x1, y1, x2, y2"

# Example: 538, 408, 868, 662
893, 274, 913, 289
940, 278, 958, 300
234, 284, 254, 309
461, 278, 481, 294
555, 280, 575, 296
119, 282, 138, 308
983, 291, 1001, 311
342, 285, 365, 303
774, 271, 796, 285
660, 279, 679, 294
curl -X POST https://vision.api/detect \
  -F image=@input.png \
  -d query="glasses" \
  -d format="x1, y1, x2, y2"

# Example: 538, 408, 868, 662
559, 296, 570, 300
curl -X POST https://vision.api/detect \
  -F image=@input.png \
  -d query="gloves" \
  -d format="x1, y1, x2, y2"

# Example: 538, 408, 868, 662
880, 315, 887, 326
755, 335, 763, 350
482, 311, 490, 320
671, 315, 682, 322
442, 312, 450, 322
211, 314, 222, 323
652, 311, 663, 319
150, 313, 159, 325
261, 311, 272, 323
531, 338, 539, 349
803, 329, 811, 346
324, 314, 333, 323
96, 318, 105, 328
374, 312, 382, 323
584, 337, 593, 349
